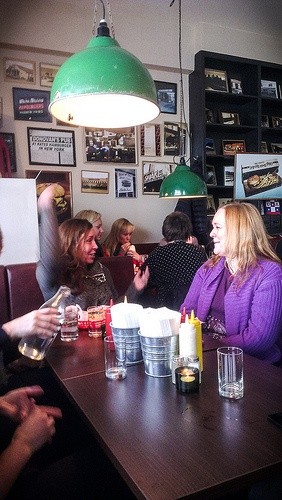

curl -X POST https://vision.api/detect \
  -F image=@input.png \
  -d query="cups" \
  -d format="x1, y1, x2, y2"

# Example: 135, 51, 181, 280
104, 336, 127, 380
87, 306, 102, 338
217, 346, 244, 400
59, 309, 79, 342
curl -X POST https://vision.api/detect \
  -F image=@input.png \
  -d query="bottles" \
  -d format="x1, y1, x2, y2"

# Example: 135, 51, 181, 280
187, 310, 203, 372
180, 307, 186, 323
105, 299, 115, 353
179, 313, 197, 362
185, 355, 201, 384
171, 355, 186, 384
18, 285, 71, 361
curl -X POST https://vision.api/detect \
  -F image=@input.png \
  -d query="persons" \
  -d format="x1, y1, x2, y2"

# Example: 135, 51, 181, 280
35, 184, 149, 311
179, 202, 282, 367
127, 211, 209, 311
74, 209, 136, 257
0, 228, 98, 500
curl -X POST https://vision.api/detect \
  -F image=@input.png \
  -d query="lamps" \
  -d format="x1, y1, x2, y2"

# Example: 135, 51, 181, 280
48, 0, 161, 130
159, 0, 208, 198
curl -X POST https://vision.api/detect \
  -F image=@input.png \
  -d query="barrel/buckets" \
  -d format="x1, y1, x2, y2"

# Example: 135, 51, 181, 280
110, 322, 143, 364
138, 330, 179, 378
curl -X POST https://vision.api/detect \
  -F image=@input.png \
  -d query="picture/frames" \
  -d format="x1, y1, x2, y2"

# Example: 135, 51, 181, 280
112, 166, 139, 199
80, 169, 111, 196
39, 61, 61, 88
0, 131, 17, 172
83, 127, 139, 167
153, 78, 178, 115
27, 126, 76, 167
11, 87, 52, 122
3, 56, 36, 85
141, 160, 177, 197
52, 116, 82, 130
205, 67, 282, 216
24, 168, 73, 226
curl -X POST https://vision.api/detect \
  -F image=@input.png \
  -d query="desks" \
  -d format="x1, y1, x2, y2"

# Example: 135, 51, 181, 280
45, 333, 282, 500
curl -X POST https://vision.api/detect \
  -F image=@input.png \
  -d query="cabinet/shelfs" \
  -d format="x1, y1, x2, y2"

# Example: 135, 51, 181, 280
188, 49, 282, 221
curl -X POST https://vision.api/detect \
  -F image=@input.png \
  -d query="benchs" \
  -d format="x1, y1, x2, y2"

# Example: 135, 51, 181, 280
0, 242, 160, 327
